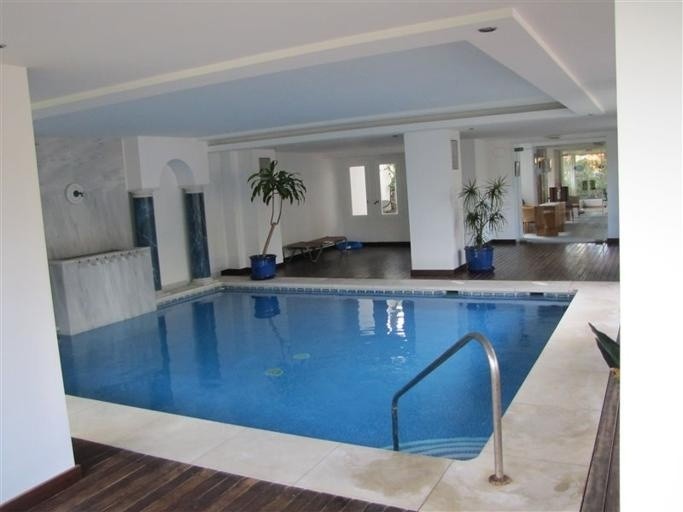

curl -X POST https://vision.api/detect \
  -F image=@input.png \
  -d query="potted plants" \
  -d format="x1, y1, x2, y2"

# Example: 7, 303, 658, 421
458, 173, 512, 274
247, 158, 308, 281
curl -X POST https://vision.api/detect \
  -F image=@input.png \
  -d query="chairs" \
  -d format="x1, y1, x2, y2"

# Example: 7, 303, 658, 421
522, 186, 604, 237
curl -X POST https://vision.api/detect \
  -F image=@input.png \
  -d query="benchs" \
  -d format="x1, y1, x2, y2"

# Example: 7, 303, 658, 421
578, 365, 620, 512
283, 235, 348, 263
